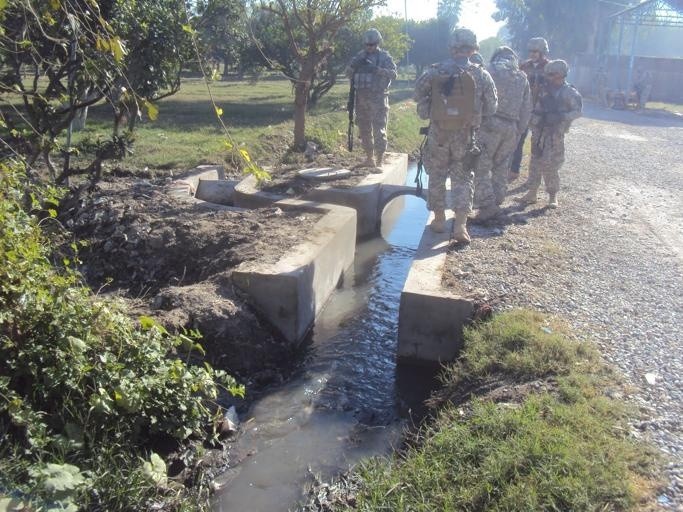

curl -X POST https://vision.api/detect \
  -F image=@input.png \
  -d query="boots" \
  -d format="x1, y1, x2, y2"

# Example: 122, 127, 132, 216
513, 189, 537, 204
548, 192, 558, 208
367, 150, 386, 173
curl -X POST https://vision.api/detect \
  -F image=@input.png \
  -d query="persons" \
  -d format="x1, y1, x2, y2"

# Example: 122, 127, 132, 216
507, 36, 551, 179
344, 27, 396, 175
470, 54, 485, 67
468, 46, 532, 223
592, 66, 611, 110
412, 28, 498, 244
631, 66, 654, 115
513, 59, 585, 210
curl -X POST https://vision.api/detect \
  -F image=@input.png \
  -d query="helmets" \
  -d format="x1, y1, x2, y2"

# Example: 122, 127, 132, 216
363, 28, 382, 46
469, 53, 483, 63
526, 38, 549, 53
490, 46, 519, 61
449, 28, 479, 49
544, 59, 569, 74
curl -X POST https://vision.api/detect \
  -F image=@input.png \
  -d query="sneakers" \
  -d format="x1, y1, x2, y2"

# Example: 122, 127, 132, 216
430, 208, 445, 233
508, 171, 520, 180
474, 190, 506, 225
454, 209, 471, 243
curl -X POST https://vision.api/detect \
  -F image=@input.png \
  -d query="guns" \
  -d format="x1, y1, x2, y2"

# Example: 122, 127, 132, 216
347, 72, 355, 151
531, 72, 557, 128
